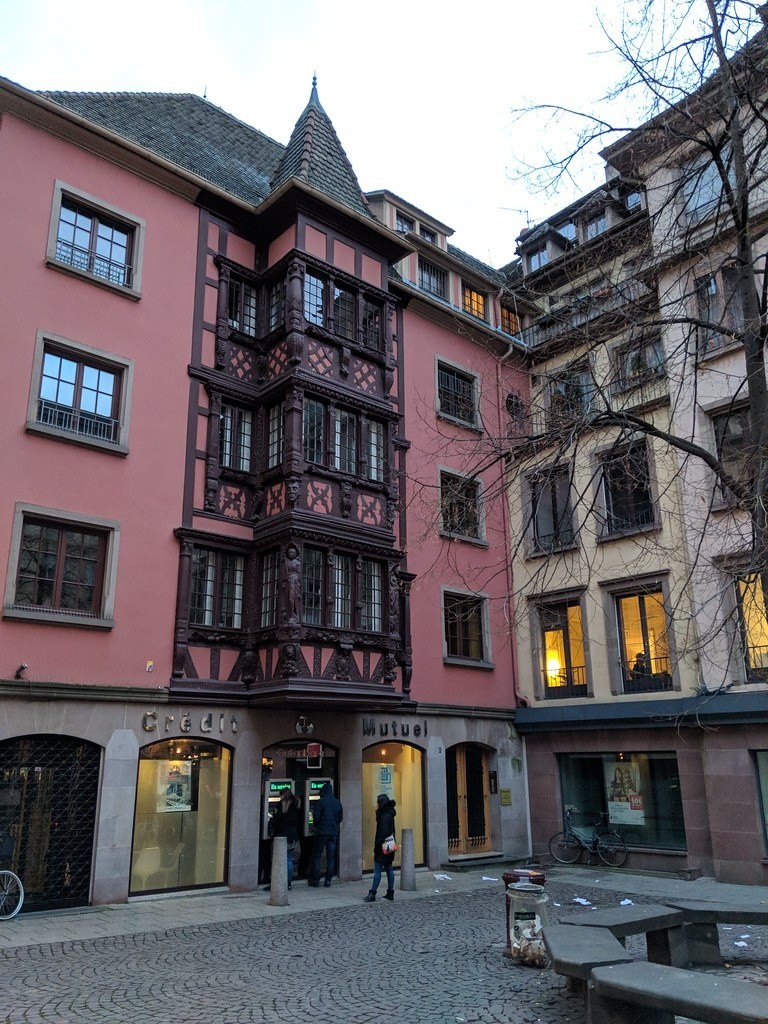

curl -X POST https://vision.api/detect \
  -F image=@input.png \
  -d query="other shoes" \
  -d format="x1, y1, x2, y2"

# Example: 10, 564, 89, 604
288, 881, 291, 890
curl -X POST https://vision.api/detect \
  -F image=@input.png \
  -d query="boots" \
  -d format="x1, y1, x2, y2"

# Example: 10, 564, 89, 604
382, 889, 395, 902
309, 873, 320, 887
324, 871, 333, 887
363, 889, 377, 901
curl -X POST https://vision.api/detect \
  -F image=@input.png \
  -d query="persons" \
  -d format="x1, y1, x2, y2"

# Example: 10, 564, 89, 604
263, 788, 301, 891
609, 766, 634, 802
630, 652, 646, 680
341, 482, 353, 516
363, 793, 397, 900
308, 784, 343, 887
278, 547, 302, 614
390, 567, 401, 615
284, 477, 302, 507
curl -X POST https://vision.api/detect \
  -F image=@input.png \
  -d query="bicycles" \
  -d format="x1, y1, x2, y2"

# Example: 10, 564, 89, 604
548, 808, 628, 867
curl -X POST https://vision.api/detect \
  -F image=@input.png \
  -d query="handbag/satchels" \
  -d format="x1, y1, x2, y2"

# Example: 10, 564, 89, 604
381, 835, 398, 854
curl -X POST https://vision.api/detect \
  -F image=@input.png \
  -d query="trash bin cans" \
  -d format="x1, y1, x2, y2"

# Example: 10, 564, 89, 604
502, 868, 547, 959
508, 882, 545, 967
